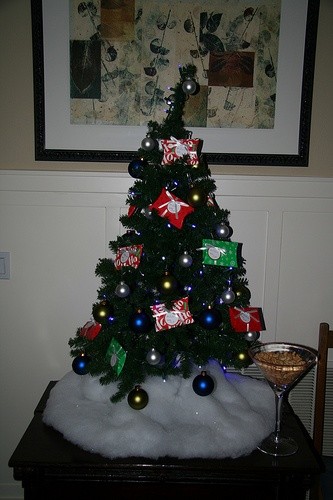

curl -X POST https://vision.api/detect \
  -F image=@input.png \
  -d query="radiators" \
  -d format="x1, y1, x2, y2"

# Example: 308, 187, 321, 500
226, 360, 333, 457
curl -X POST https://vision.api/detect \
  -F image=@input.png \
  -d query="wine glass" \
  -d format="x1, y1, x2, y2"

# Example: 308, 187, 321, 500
247, 341, 317, 457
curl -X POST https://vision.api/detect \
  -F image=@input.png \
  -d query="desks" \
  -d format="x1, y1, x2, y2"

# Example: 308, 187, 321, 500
8, 381, 326, 500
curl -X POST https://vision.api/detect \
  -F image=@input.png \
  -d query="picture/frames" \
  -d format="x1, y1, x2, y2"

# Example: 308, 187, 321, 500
30, 0, 321, 167
